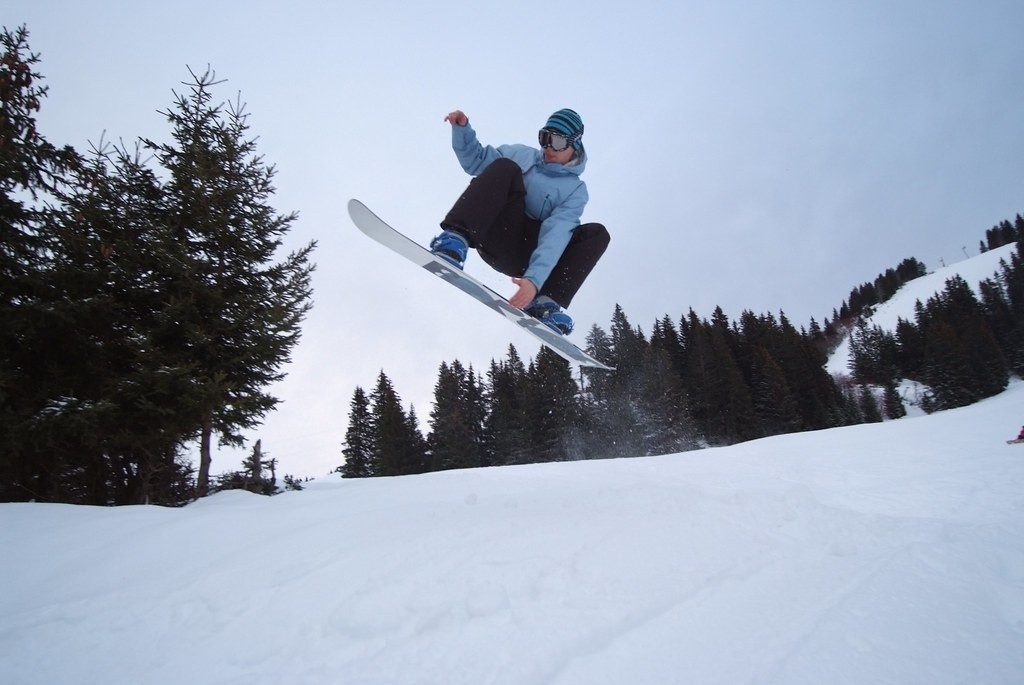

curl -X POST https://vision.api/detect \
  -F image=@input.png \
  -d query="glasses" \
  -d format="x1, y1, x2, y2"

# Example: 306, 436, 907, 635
539, 126, 584, 151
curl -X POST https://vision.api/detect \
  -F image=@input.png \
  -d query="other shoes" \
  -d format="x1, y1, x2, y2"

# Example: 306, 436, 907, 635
524, 294, 574, 335
430, 230, 467, 269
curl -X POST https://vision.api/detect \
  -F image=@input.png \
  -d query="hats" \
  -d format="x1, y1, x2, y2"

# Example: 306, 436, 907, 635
545, 109, 584, 150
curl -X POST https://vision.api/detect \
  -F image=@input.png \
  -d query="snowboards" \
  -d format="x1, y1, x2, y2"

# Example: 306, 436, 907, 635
346, 198, 613, 372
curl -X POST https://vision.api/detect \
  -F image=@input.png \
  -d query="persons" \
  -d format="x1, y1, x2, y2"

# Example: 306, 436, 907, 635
429, 108, 611, 336
